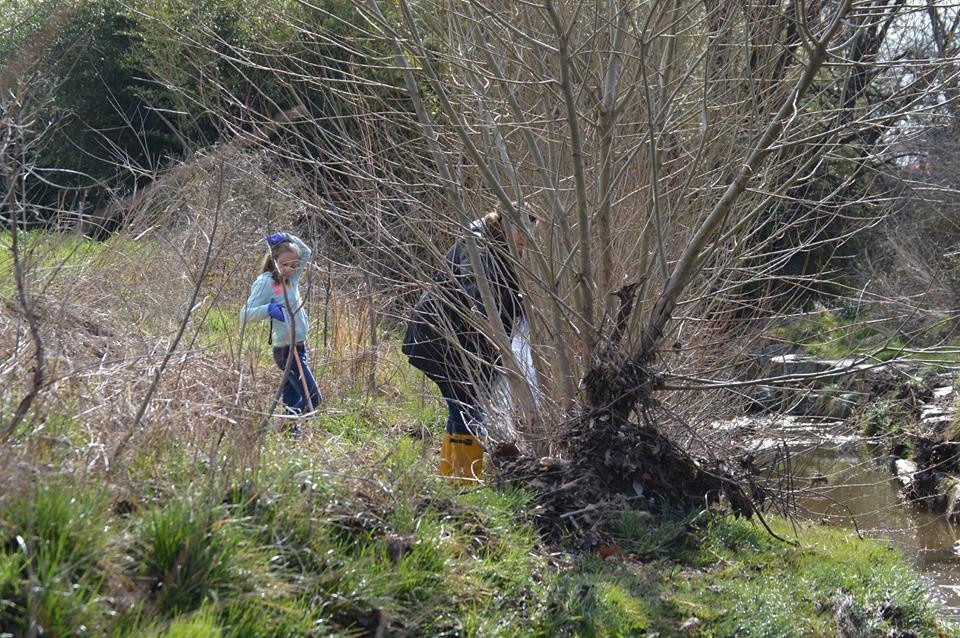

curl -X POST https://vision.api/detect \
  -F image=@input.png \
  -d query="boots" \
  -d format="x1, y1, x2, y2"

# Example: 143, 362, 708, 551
438, 432, 454, 486
449, 433, 484, 489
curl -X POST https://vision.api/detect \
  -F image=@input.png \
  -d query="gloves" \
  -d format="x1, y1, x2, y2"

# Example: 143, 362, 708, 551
267, 232, 286, 246
268, 303, 286, 322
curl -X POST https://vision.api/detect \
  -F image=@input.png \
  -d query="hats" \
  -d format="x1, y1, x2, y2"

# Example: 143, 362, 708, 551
498, 201, 538, 224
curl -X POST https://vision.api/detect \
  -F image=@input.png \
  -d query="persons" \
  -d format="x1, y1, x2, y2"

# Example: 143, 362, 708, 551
240, 233, 322, 430
403, 201, 541, 490
